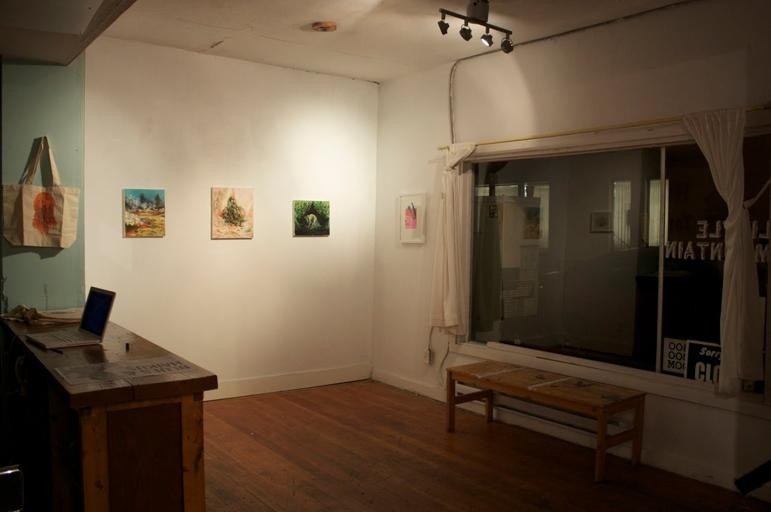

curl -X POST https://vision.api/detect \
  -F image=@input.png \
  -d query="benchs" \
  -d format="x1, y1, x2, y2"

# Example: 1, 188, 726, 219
446, 358, 648, 482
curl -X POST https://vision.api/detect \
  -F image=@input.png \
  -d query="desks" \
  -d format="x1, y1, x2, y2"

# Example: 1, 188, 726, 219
0, 307, 219, 512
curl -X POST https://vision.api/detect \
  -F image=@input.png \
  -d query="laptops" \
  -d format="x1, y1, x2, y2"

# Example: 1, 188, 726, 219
25, 286, 116, 351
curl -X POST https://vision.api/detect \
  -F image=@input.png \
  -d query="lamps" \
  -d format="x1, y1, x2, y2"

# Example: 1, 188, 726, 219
438, 1, 514, 54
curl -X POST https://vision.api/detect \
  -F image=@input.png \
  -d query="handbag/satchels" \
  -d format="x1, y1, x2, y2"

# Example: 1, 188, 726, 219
0, 135, 82, 250
